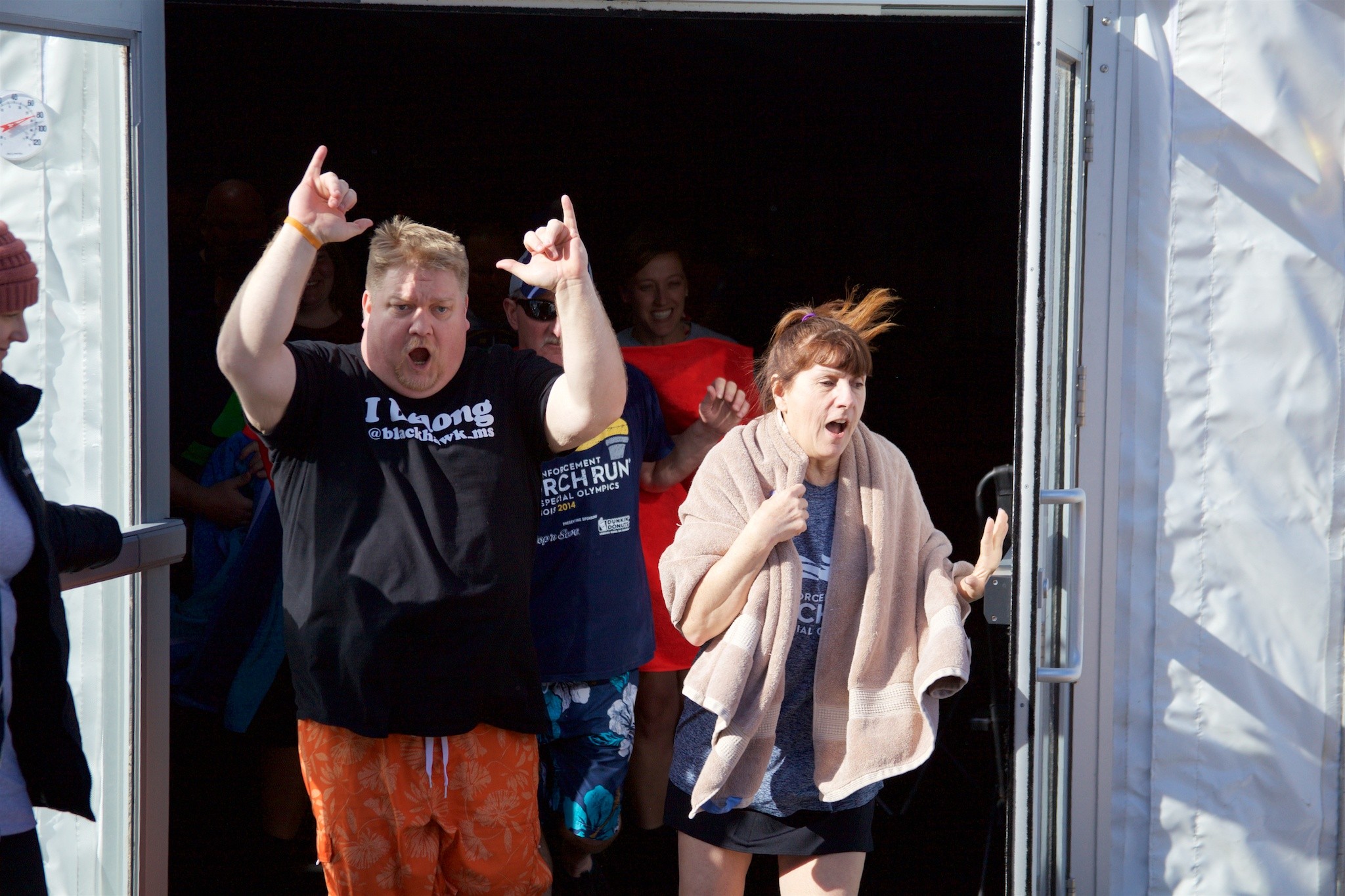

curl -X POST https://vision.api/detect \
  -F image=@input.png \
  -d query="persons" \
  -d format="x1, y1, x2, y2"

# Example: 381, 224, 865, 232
615, 244, 763, 896
215, 143, 629, 896
0, 220, 124, 896
167, 175, 524, 896
504, 249, 750, 896
657, 288, 1011, 896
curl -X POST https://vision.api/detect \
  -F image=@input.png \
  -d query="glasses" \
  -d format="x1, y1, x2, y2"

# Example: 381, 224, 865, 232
502, 292, 557, 321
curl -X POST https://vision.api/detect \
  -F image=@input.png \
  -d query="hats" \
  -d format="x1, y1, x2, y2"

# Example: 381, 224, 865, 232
0, 219, 38, 312
508, 245, 594, 298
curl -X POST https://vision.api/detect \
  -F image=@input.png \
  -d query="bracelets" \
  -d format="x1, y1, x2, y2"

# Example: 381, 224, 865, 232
283, 215, 322, 250
